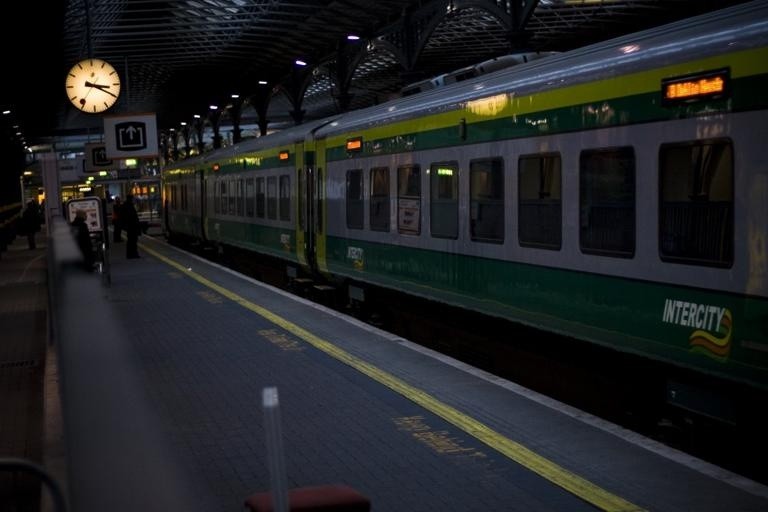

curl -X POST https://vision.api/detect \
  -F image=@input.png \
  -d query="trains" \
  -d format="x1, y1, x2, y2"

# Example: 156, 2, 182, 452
93, 0, 768, 426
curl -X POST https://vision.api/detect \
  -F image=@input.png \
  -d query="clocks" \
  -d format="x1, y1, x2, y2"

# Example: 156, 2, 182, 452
66, 59, 121, 114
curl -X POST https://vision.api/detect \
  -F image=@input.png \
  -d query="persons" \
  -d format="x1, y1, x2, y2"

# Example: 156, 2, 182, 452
111, 196, 124, 242
22, 201, 40, 250
122, 194, 142, 259
69, 210, 94, 274
